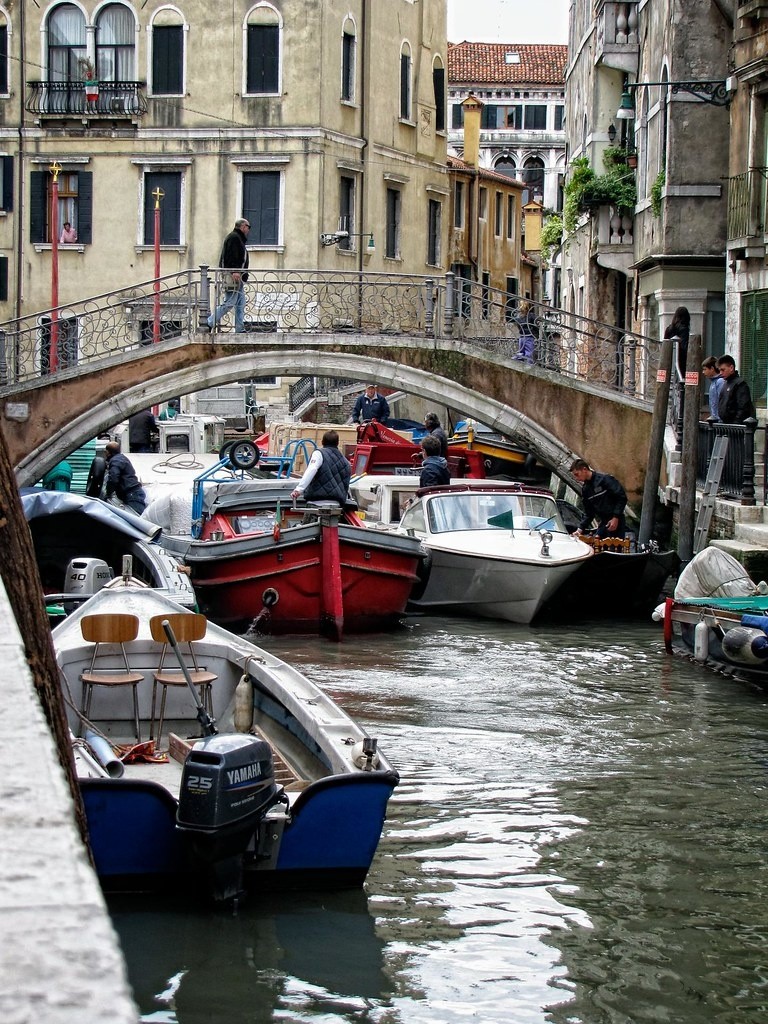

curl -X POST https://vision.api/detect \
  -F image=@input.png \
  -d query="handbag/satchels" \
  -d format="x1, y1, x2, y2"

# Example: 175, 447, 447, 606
222, 272, 241, 292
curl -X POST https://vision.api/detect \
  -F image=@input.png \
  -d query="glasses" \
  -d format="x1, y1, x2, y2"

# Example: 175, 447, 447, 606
245, 225, 251, 229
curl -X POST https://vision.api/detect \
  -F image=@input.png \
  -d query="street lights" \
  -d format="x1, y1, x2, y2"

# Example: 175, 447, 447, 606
48, 159, 63, 374
150, 187, 165, 341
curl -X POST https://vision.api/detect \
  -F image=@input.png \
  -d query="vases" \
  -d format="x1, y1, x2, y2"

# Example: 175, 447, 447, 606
626, 156, 637, 169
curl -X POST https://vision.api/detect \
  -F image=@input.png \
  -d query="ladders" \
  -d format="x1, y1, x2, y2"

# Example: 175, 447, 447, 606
169, 724, 304, 787
693, 435, 728, 555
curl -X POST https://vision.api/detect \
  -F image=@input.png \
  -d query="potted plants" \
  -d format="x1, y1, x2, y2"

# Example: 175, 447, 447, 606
76, 56, 100, 101
603, 147, 636, 164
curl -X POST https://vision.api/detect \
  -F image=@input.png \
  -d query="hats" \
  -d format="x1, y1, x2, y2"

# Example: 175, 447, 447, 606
366, 383, 375, 389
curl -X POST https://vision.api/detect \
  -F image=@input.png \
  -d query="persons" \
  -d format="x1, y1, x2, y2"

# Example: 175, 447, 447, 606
510, 301, 537, 366
290, 429, 351, 504
59, 222, 77, 243
411, 412, 448, 459
352, 383, 391, 425
665, 306, 691, 379
129, 407, 159, 453
569, 459, 628, 551
207, 218, 252, 333
402, 435, 450, 510
703, 355, 755, 424
105, 441, 146, 514
159, 399, 178, 421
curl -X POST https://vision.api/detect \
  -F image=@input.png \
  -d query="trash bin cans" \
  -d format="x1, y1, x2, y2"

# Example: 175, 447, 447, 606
254, 415, 265, 435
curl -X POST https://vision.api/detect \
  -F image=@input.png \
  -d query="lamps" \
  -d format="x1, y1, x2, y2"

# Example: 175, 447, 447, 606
320, 230, 376, 255
615, 73, 738, 120
607, 123, 617, 142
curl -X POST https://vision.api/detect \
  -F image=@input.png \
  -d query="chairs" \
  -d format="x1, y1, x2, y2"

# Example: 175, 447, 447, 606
149, 613, 220, 743
577, 531, 631, 555
77, 613, 146, 746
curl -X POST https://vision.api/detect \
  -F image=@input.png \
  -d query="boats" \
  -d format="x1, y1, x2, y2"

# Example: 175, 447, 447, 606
14, 488, 200, 626
52, 554, 400, 895
142, 416, 681, 641
